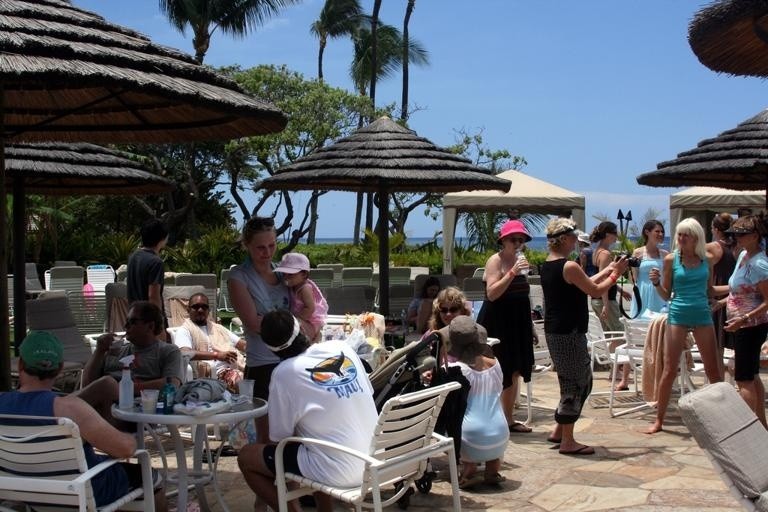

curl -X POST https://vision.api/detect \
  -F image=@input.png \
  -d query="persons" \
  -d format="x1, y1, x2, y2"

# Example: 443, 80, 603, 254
575, 234, 591, 269
0, 332, 165, 511
541, 220, 628, 456
445, 317, 508, 488
613, 221, 671, 391
70, 304, 181, 428
273, 253, 327, 344
408, 274, 440, 333
710, 218, 767, 430
421, 287, 473, 384
650, 218, 723, 424
125, 220, 169, 337
588, 221, 632, 380
237, 309, 384, 512
228, 218, 289, 442
705, 214, 743, 285
476, 220, 538, 433
173, 291, 246, 393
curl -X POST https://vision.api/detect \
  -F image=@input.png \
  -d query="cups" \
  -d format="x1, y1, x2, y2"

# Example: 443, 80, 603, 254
139, 389, 160, 414
238, 379, 255, 402
109, 336, 123, 356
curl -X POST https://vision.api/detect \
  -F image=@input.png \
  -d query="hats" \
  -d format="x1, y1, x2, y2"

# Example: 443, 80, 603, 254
722, 226, 754, 236
18, 330, 65, 370
271, 254, 311, 274
439, 315, 495, 359
497, 221, 533, 245
264, 310, 302, 352
577, 233, 591, 245
547, 224, 583, 238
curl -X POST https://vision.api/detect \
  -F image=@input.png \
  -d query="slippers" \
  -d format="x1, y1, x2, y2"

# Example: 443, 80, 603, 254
613, 384, 630, 391
509, 423, 532, 434
458, 473, 486, 488
547, 438, 562, 443
558, 445, 595, 455
484, 473, 505, 485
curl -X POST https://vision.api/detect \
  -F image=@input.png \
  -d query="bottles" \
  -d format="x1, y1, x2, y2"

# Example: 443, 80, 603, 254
161, 376, 177, 414
514, 247, 530, 275
229, 339, 238, 372
400, 307, 408, 336
155, 393, 165, 414
325, 326, 332, 341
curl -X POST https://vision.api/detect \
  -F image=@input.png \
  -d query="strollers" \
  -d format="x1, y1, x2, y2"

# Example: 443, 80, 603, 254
368, 332, 442, 510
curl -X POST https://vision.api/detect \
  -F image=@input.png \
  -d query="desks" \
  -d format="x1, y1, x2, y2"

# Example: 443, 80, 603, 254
111, 396, 269, 512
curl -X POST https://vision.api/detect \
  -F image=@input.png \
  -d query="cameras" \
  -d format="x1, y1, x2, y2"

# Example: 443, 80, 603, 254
614, 251, 641, 267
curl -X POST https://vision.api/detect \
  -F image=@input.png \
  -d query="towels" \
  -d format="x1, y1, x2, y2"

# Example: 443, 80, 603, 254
181, 317, 246, 378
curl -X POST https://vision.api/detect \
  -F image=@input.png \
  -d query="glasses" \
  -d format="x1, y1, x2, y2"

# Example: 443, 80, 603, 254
439, 306, 463, 314
607, 232, 617, 235
503, 238, 524, 243
189, 304, 210, 310
126, 316, 146, 325
245, 217, 274, 236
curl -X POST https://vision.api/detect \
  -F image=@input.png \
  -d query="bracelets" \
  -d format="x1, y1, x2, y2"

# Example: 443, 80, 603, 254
652, 280, 661, 286
743, 314, 749, 323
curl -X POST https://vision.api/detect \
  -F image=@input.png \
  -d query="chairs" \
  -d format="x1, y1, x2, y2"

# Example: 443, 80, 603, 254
274, 382, 462, 512
106, 282, 131, 331
86, 264, 115, 291
175, 274, 217, 322
24, 262, 45, 299
585, 313, 628, 381
6, 276, 14, 333
162, 284, 214, 326
44, 268, 51, 292
66, 292, 110, 334
676, 380, 767, 512
218, 269, 237, 318
514, 320, 560, 424
23, 296, 93, 363
49, 267, 84, 292
0, 413, 155, 512
608, 317, 709, 419
55, 260, 77, 266
10, 355, 84, 397
307, 263, 487, 340
